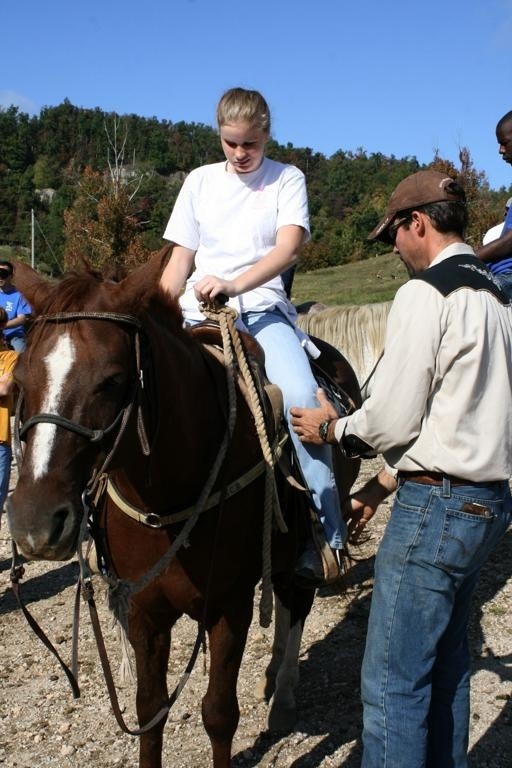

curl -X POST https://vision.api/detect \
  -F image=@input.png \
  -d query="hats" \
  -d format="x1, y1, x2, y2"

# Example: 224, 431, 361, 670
1, 262, 13, 272
367, 171, 462, 241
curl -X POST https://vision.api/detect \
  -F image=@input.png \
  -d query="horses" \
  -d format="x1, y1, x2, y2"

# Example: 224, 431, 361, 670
6, 242, 363, 768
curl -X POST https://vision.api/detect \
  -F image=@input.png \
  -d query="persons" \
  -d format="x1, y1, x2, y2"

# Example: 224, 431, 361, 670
1, 262, 31, 352
290, 170, 510, 768
159, 89, 347, 587
0, 314, 22, 520
476, 108, 512, 294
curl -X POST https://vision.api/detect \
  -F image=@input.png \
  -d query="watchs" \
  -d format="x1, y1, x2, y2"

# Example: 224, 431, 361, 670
318, 418, 334, 445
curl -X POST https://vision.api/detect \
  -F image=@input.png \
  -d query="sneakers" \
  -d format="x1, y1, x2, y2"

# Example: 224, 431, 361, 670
295, 547, 349, 581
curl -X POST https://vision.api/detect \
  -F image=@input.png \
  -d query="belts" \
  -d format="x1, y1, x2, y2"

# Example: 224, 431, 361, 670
399, 471, 466, 488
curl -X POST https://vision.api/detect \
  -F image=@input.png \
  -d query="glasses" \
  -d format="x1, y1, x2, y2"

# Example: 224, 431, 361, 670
388, 220, 406, 241
